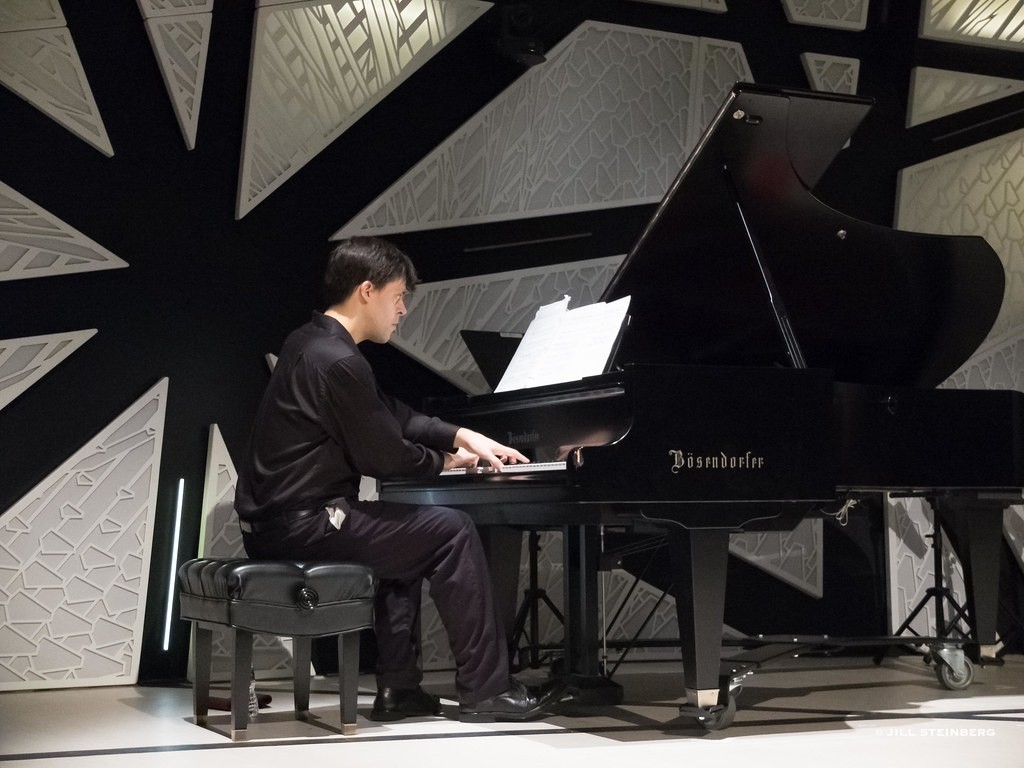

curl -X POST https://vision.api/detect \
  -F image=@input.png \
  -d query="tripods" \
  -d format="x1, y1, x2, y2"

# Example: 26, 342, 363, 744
870, 503, 971, 666
506, 526, 566, 674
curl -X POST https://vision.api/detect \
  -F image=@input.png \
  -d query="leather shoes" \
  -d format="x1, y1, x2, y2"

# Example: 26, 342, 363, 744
371, 688, 442, 721
458, 682, 568, 722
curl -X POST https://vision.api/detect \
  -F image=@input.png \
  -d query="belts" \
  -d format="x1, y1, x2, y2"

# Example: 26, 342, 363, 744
238, 505, 317, 534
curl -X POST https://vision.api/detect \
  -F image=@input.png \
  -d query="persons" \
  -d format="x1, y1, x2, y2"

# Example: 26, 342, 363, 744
231, 235, 567, 725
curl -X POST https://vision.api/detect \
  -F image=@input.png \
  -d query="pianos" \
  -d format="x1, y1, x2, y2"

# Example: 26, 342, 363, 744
379, 80, 1024, 731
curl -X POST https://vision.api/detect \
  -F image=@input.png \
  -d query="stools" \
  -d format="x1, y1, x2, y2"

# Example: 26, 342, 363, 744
178, 556, 376, 741
